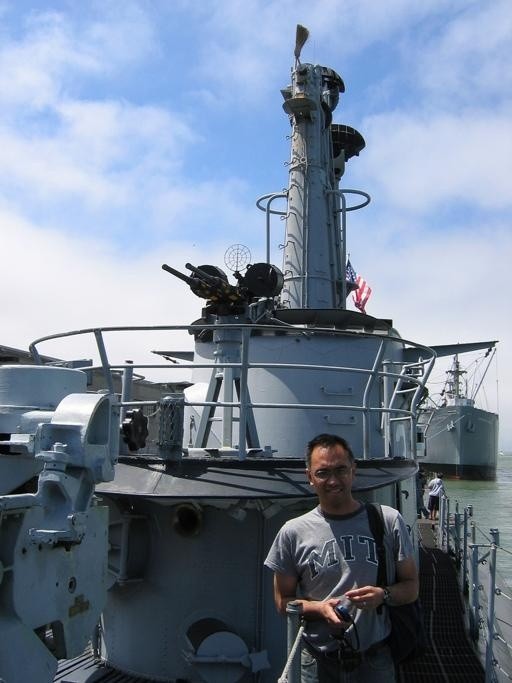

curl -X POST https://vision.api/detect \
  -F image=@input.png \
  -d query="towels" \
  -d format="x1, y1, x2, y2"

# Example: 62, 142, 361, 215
419, 519, 439, 548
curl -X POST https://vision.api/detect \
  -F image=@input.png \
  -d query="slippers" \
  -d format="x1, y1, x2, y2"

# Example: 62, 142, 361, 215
366, 502, 430, 664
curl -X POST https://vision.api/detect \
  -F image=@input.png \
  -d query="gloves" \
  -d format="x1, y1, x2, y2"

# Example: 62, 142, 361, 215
416, 347, 499, 481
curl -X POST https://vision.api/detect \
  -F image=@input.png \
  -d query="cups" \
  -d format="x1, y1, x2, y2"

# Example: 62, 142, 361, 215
309, 464, 353, 480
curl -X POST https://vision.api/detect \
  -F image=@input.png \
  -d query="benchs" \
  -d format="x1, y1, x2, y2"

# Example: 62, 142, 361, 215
343, 258, 374, 314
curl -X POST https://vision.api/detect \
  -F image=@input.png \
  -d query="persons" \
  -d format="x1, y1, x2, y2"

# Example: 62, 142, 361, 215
428, 471, 448, 520
415, 462, 431, 520
262, 432, 421, 680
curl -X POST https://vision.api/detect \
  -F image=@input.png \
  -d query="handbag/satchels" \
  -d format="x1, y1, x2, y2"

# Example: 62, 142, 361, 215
382, 585, 392, 605
